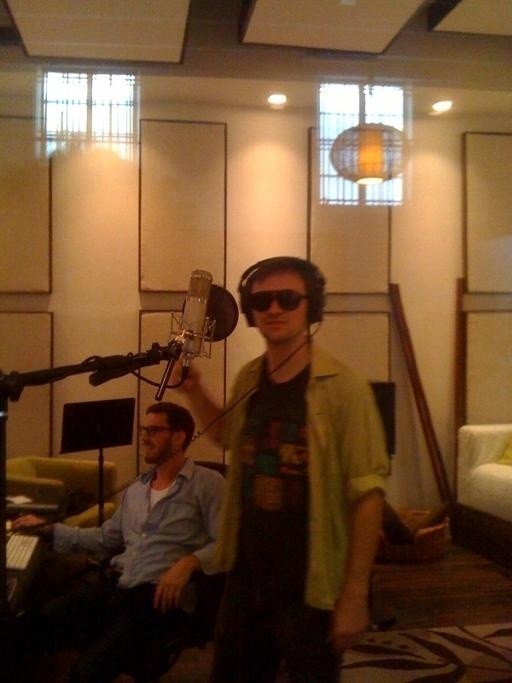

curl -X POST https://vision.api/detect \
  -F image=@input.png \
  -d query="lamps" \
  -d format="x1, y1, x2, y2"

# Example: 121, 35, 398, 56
330, 123, 411, 185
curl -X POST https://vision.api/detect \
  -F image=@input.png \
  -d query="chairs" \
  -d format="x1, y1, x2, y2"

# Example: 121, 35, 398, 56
5, 454, 237, 682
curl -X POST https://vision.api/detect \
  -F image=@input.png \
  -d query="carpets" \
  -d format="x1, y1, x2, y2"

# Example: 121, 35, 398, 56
338, 621, 512, 683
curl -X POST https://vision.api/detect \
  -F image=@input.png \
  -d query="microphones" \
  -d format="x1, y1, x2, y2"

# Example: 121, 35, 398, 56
178, 269, 214, 381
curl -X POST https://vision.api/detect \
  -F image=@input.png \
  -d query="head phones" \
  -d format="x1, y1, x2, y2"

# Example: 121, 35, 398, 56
237, 255, 328, 325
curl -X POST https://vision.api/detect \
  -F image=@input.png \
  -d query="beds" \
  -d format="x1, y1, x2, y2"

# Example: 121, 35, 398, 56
447, 421, 511, 570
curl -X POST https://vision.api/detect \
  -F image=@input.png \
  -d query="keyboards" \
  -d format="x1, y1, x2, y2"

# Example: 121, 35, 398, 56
5, 533, 40, 571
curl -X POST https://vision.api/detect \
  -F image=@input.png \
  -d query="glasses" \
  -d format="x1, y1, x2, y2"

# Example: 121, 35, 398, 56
248, 288, 310, 312
138, 424, 172, 437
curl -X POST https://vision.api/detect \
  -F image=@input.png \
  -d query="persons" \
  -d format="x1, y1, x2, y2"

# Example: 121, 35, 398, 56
12, 401, 230, 683
173, 256, 391, 682
